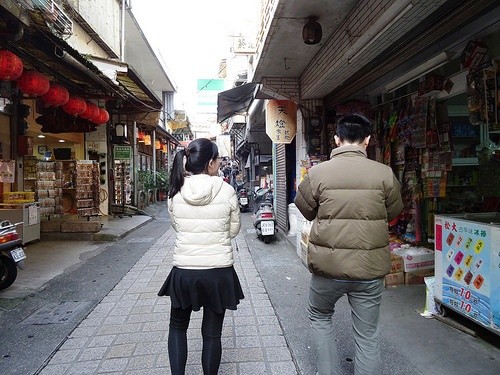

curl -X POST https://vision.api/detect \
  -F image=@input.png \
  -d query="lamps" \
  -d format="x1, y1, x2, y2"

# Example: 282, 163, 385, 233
143, 130, 152, 146
155, 136, 160, 150
301, 17, 323, 45
37, 133, 46, 138
384, 51, 454, 93
162, 140, 167, 153
114, 113, 128, 139
58, 138, 66, 143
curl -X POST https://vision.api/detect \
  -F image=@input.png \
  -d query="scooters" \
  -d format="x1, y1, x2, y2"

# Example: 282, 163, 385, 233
249, 166, 279, 243
232, 175, 249, 213
0, 218, 26, 291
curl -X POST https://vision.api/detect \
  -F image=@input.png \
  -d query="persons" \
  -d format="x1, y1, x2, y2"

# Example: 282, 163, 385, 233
157, 138, 245, 375
294, 113, 404, 375
216, 158, 238, 179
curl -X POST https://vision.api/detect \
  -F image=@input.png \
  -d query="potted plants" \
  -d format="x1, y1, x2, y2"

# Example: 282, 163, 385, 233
139, 166, 169, 204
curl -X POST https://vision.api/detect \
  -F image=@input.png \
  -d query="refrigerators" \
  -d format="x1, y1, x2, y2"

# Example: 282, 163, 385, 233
434, 211, 499, 336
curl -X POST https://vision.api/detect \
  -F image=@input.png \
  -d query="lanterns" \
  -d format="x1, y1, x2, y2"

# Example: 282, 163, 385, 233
0, 49, 109, 126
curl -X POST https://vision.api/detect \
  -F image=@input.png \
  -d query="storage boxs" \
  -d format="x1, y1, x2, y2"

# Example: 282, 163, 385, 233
407, 246, 435, 270
296, 230, 309, 267
383, 271, 405, 289
388, 252, 404, 274
393, 248, 419, 272
404, 268, 434, 285
